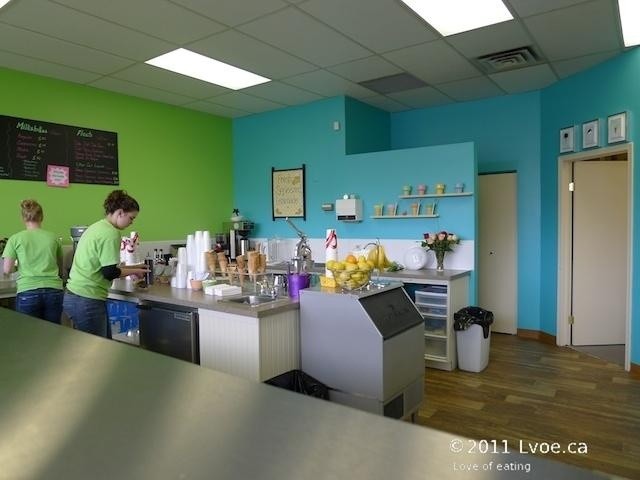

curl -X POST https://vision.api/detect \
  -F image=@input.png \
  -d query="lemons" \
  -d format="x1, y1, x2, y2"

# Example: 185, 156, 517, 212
326, 255, 374, 288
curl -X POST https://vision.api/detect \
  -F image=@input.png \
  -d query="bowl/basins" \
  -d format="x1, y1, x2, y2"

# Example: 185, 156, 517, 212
189, 279, 216, 291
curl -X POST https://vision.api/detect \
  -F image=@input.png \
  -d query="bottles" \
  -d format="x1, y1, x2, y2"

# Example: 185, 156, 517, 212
153, 248, 164, 260
144, 251, 153, 285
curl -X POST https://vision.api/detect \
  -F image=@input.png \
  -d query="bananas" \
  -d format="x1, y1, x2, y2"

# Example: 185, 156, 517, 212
368, 246, 391, 272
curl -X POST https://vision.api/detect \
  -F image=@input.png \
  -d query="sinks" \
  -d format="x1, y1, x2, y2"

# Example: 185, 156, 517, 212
229, 294, 277, 305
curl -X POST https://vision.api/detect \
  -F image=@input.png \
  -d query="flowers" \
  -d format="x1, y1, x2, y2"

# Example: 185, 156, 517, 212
416, 231, 464, 264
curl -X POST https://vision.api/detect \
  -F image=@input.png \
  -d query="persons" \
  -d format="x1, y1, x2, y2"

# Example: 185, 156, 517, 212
1, 199, 64, 325
62, 190, 151, 339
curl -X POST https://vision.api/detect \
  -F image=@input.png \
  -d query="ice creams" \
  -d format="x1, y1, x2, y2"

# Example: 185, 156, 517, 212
208, 252, 266, 286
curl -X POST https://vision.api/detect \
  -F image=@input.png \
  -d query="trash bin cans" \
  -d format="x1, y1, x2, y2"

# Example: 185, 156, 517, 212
455, 306, 493, 373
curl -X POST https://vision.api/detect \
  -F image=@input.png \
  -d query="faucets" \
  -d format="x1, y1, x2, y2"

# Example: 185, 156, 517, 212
256, 281, 264, 289
363, 242, 381, 268
263, 279, 270, 288
284, 216, 316, 269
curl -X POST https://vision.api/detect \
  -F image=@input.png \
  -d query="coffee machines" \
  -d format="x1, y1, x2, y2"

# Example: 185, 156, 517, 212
222, 207, 256, 262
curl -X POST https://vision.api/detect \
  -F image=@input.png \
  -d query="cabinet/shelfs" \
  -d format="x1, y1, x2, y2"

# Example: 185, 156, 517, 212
139, 308, 196, 364
371, 268, 471, 371
370, 192, 473, 218
299, 281, 425, 420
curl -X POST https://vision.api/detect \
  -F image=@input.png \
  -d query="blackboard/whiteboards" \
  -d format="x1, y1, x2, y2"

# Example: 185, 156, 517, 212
0, 115, 119, 185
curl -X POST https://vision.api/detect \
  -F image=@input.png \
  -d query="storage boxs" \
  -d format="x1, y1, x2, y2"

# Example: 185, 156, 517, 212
424, 337, 448, 359
423, 316, 447, 337
415, 286, 447, 304
415, 305, 447, 316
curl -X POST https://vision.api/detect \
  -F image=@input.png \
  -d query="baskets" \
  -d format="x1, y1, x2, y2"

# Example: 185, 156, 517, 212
333, 269, 371, 292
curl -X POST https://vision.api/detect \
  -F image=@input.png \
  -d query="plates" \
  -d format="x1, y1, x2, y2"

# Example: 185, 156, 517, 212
364, 242, 377, 255
403, 247, 429, 271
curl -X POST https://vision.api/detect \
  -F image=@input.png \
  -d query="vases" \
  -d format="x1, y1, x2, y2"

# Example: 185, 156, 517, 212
435, 251, 445, 270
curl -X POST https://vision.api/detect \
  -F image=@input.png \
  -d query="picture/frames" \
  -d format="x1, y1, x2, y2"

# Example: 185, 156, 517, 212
559, 126, 575, 154
608, 111, 628, 144
582, 117, 600, 150
272, 164, 306, 220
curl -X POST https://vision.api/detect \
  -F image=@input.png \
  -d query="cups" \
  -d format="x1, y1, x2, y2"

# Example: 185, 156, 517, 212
403, 183, 465, 195
112, 231, 141, 291
324, 228, 338, 278
170, 230, 210, 289
206, 251, 265, 287
373, 204, 435, 217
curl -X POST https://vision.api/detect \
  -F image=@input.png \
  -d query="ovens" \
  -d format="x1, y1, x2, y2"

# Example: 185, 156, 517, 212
135, 301, 197, 366
